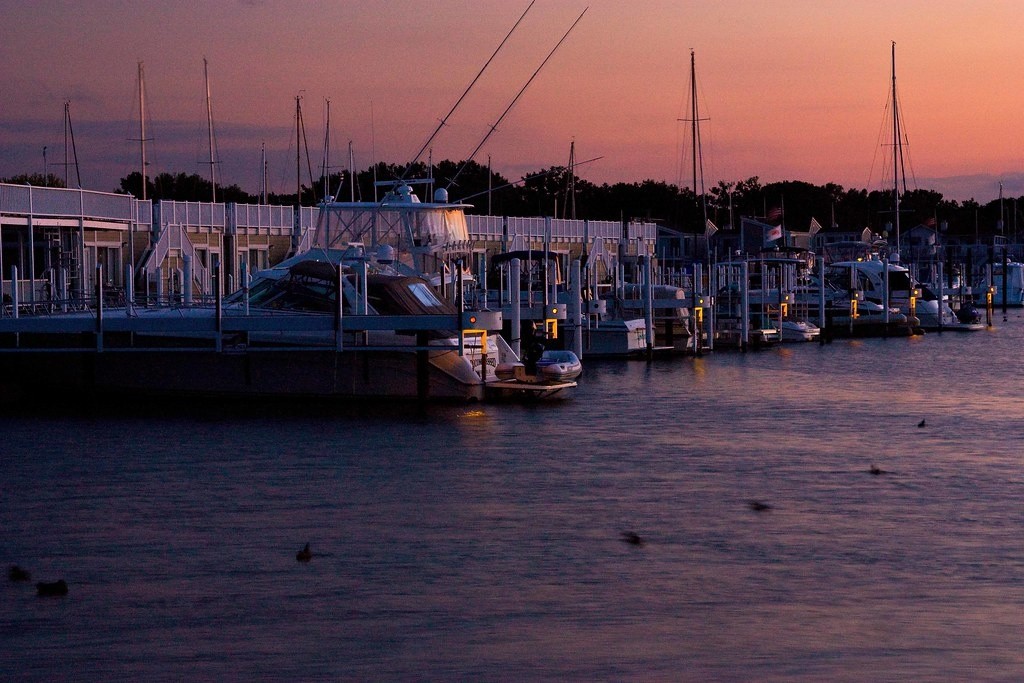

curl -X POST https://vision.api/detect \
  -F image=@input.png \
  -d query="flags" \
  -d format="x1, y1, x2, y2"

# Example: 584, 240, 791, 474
704, 219, 718, 241
767, 225, 782, 242
809, 216, 821, 239
923, 218, 936, 226
862, 227, 872, 242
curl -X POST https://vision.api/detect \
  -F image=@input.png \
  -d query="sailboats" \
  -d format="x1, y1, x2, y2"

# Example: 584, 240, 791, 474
220, 41, 1024, 402
